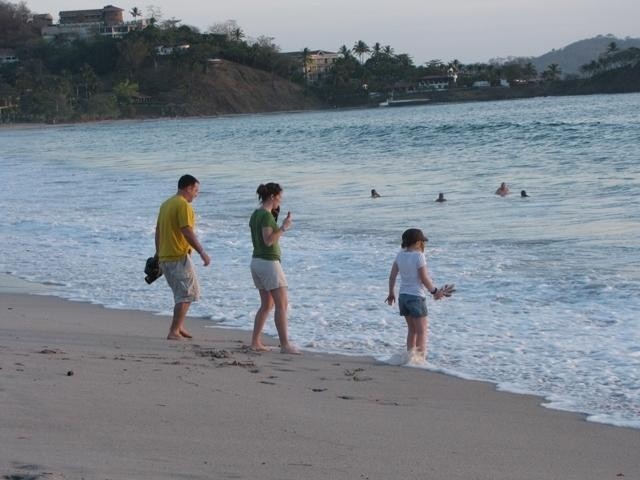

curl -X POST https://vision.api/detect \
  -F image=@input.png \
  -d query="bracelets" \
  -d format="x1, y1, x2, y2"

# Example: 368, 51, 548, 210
281, 225, 286, 233
431, 288, 438, 293
201, 250, 208, 256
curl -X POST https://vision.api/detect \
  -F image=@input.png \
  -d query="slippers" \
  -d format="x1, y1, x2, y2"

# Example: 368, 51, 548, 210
273, 206, 280, 223
144, 258, 163, 284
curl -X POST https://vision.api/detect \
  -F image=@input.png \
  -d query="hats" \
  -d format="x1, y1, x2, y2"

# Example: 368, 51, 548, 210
402, 229, 428, 242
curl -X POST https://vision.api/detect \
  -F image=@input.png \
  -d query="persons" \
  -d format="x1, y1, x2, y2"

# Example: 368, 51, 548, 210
384, 229, 446, 363
435, 192, 448, 202
371, 189, 380, 197
247, 182, 303, 355
494, 182, 511, 198
520, 190, 530, 198
155, 175, 213, 341
